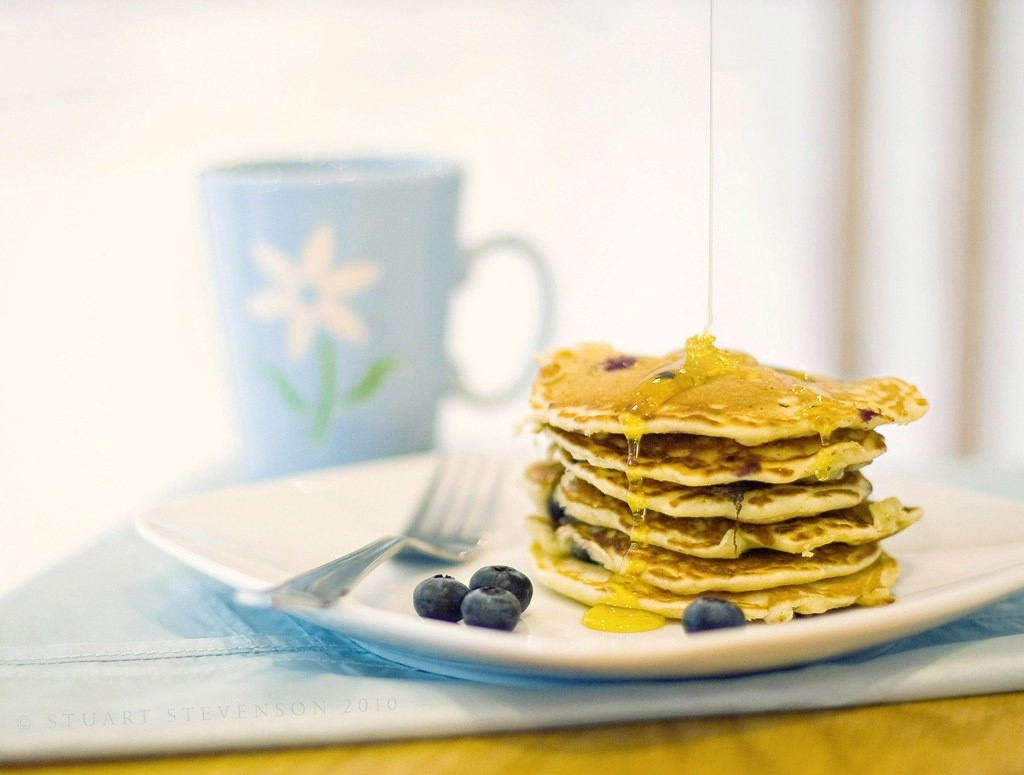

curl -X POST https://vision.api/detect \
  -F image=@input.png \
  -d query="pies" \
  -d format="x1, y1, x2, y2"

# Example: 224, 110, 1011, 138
527, 354, 928, 618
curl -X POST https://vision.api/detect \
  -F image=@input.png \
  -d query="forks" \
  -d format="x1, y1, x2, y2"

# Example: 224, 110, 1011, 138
271, 462, 508, 613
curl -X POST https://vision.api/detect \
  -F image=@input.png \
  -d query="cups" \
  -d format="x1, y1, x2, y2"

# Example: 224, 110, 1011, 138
204, 160, 556, 486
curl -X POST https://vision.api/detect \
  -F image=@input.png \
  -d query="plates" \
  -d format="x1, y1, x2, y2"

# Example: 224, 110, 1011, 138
135, 450, 1024, 682
